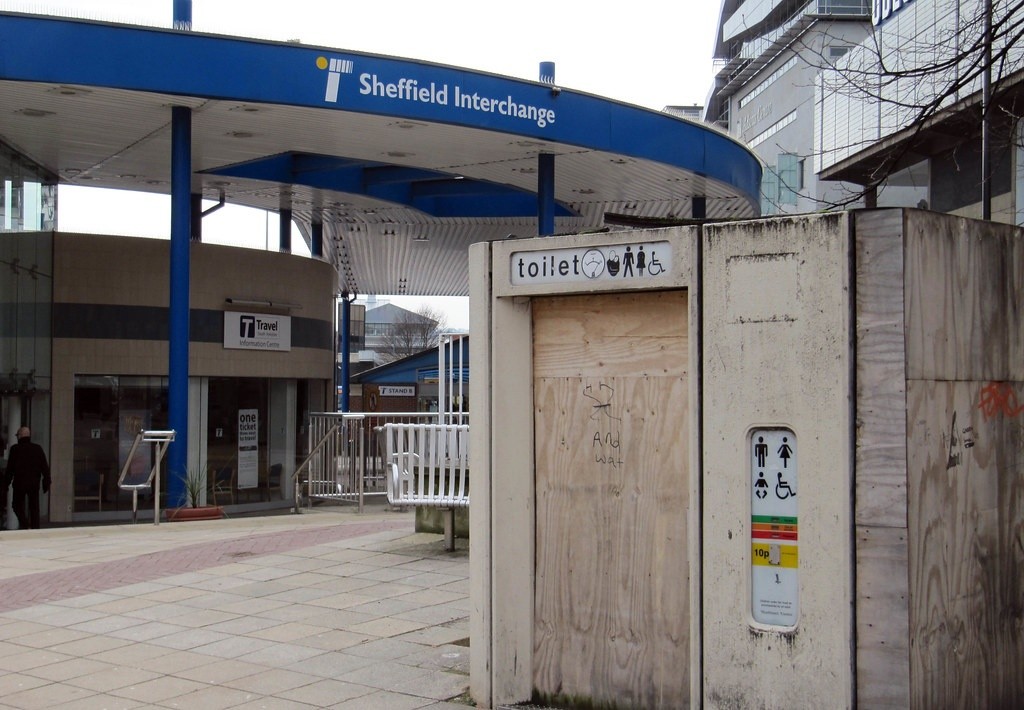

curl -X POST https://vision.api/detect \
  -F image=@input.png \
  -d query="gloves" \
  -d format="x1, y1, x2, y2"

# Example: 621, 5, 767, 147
42, 477, 51, 494
2, 478, 12, 491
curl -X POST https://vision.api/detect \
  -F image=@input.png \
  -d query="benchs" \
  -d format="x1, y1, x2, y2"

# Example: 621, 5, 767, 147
383, 424, 470, 552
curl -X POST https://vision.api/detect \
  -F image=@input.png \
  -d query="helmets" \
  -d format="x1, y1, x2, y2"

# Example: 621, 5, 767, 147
16, 426, 30, 440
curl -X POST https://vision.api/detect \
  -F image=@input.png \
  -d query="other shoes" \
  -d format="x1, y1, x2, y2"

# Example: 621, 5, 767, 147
30, 519, 40, 529
18, 520, 29, 529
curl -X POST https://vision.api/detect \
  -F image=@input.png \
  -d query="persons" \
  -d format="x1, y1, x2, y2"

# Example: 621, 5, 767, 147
1, 427, 51, 529
